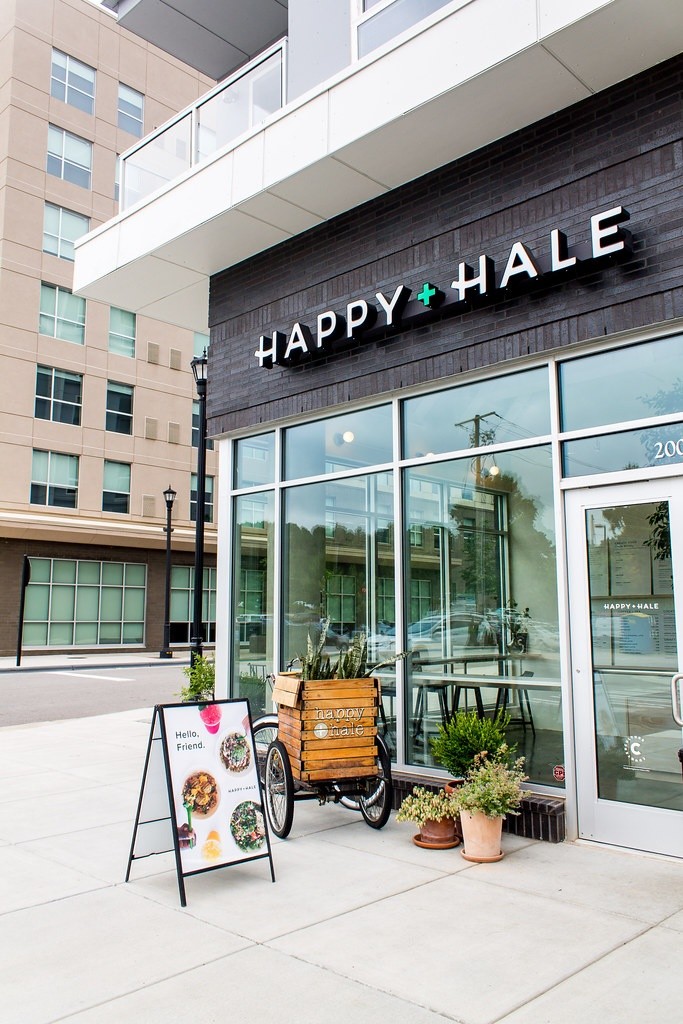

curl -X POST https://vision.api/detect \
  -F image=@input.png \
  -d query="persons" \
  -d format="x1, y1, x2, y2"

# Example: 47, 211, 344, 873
465, 617, 478, 649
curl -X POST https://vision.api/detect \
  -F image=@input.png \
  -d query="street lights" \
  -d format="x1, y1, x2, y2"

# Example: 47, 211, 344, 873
159, 484, 177, 657
188, 347, 208, 702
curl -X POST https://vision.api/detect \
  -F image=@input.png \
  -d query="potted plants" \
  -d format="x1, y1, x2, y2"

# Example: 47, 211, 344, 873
431, 706, 520, 797
171, 651, 267, 723
395, 783, 460, 848
448, 744, 529, 863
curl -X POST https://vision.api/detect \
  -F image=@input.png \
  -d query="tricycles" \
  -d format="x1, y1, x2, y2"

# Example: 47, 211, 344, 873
251, 656, 393, 839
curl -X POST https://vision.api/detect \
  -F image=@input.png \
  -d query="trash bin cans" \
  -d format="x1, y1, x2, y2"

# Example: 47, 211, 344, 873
621, 613, 653, 655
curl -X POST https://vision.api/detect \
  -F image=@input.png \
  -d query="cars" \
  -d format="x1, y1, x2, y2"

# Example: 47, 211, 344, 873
367, 612, 498, 660
336, 625, 392, 650
283, 622, 339, 646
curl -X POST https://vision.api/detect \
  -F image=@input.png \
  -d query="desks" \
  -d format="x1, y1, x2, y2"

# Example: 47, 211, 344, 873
363, 652, 562, 778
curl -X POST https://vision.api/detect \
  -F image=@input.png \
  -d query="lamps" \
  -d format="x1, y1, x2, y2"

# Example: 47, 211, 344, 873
417, 452, 433, 458
482, 466, 499, 478
333, 431, 355, 447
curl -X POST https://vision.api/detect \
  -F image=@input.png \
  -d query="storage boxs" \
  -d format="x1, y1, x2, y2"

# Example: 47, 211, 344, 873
271, 671, 379, 781
